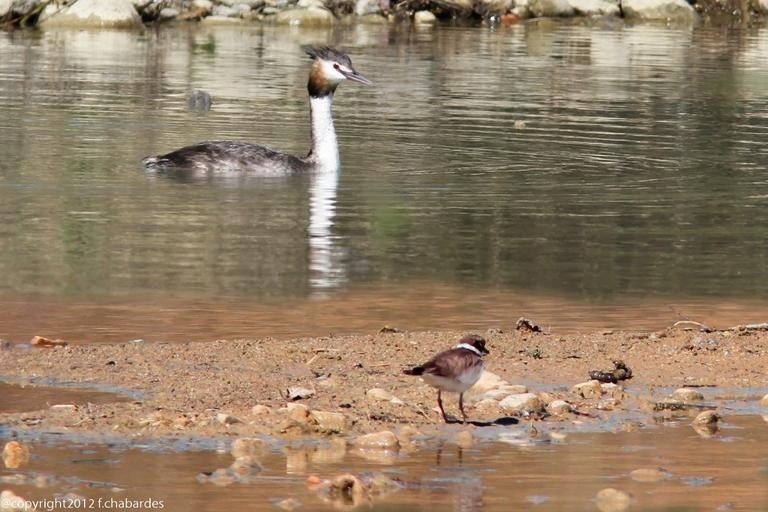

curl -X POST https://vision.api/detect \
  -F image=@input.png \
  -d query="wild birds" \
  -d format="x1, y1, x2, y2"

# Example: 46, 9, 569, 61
403, 333, 490, 422
142, 44, 373, 173
